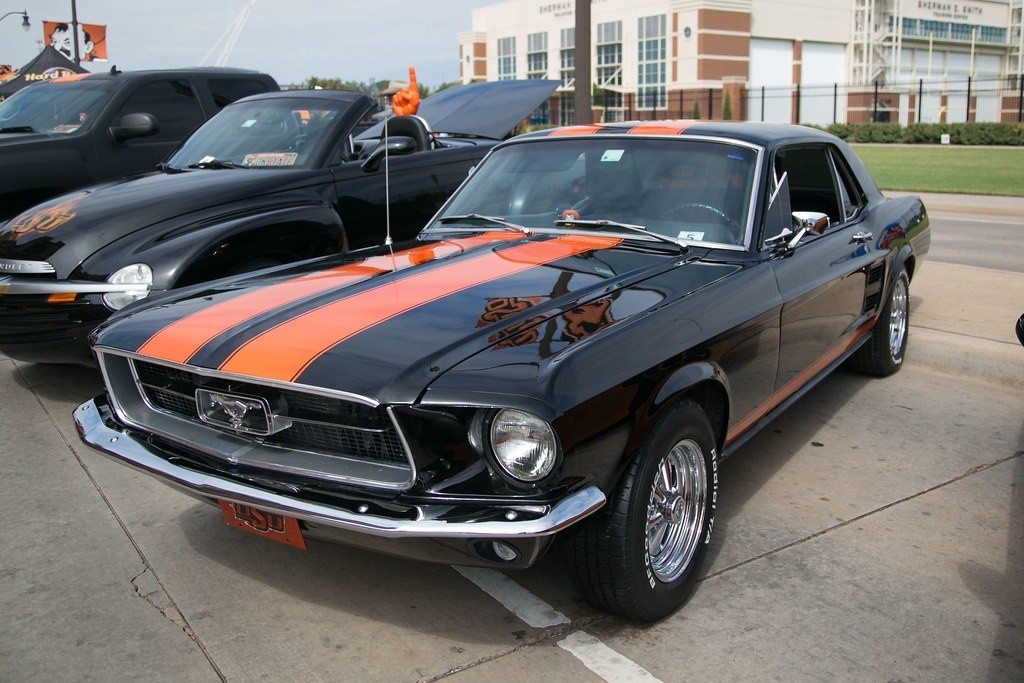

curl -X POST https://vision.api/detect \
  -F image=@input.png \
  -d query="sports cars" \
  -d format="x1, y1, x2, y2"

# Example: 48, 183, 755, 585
0, 78, 567, 373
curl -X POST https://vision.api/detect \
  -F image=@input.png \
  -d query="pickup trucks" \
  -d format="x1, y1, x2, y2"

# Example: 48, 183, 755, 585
0, 66, 379, 221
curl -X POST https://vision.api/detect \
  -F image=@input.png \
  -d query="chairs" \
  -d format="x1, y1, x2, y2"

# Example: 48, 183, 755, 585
360, 115, 434, 160
296, 117, 350, 166
576, 151, 638, 216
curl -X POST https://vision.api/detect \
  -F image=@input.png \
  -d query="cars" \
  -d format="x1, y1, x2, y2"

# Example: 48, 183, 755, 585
70, 119, 931, 627
